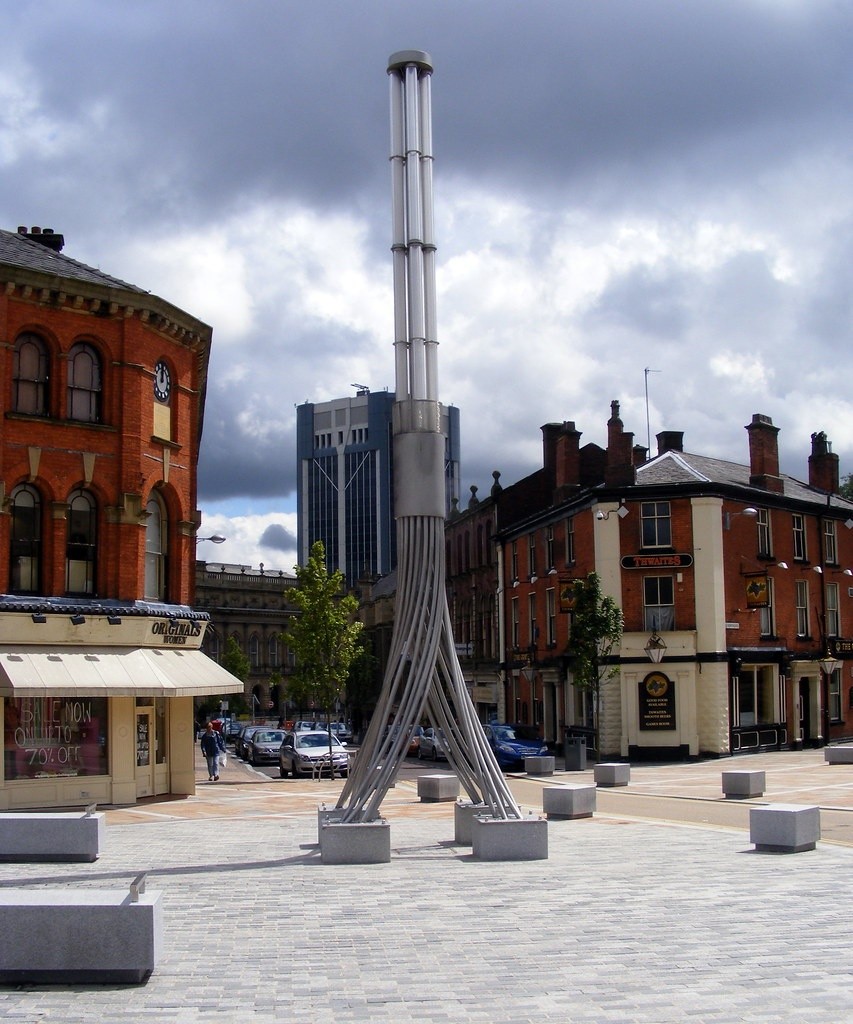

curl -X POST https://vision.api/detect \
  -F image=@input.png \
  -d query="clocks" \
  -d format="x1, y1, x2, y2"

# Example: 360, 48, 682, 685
152, 361, 171, 401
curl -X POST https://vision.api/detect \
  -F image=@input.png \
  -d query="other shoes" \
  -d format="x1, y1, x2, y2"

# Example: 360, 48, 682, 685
209, 777, 219, 781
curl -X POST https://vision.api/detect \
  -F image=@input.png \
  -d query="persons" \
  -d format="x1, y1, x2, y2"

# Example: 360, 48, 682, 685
194, 716, 200, 745
200, 722, 227, 781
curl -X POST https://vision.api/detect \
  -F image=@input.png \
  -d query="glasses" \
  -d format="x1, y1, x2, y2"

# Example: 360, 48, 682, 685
207, 726, 211, 727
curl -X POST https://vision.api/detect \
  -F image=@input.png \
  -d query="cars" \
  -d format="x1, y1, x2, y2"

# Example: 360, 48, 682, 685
380, 725, 401, 753
420, 727, 453, 761
281, 719, 351, 737
280, 730, 351, 779
249, 729, 287, 764
235, 725, 272, 761
480, 723, 548, 770
400, 724, 424, 754
221, 722, 242, 743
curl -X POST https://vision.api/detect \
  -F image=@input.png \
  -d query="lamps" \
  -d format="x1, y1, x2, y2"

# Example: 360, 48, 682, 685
644, 626, 667, 665
166, 612, 180, 627
724, 508, 758, 529
70, 607, 86, 626
31, 604, 47, 625
832, 568, 852, 576
530, 576, 550, 584
520, 656, 539, 681
187, 615, 201, 629
818, 646, 839, 676
548, 569, 571, 575
107, 608, 122, 625
196, 534, 226, 546
766, 562, 788, 570
801, 566, 823, 573
513, 581, 531, 588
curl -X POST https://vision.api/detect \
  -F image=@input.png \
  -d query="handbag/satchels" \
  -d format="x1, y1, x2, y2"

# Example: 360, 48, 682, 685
219, 752, 227, 767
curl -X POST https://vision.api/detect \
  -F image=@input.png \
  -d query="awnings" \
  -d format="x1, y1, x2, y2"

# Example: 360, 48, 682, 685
0, 643, 244, 697
